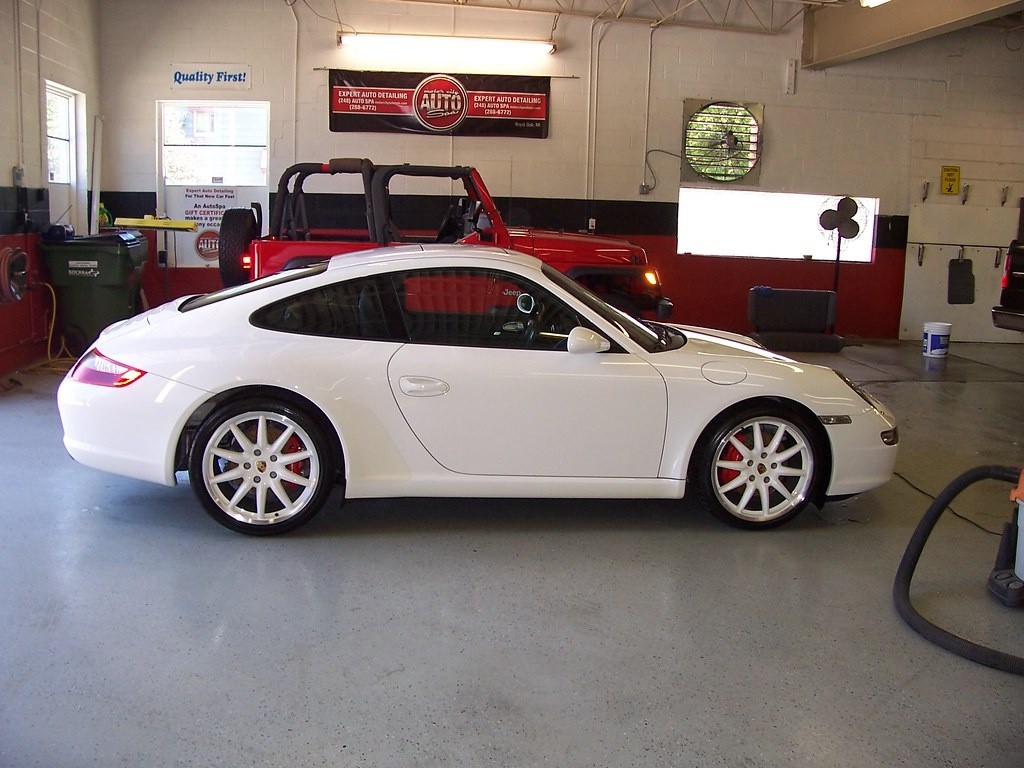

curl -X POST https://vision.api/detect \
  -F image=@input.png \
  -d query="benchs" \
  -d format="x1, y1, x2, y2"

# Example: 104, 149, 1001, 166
287, 279, 357, 337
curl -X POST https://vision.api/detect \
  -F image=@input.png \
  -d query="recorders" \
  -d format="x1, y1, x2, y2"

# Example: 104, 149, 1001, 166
47, 205, 75, 240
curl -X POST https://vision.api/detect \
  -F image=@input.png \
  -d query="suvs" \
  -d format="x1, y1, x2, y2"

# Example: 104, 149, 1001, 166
217, 159, 676, 324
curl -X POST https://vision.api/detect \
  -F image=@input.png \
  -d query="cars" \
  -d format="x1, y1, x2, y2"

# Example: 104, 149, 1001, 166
56, 240, 905, 538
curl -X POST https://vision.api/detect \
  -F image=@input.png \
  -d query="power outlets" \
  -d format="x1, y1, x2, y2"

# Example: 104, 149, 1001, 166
639, 184, 650, 194
589, 219, 596, 230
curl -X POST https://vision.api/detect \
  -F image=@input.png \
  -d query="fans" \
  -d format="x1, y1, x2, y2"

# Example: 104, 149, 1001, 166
818, 192, 868, 290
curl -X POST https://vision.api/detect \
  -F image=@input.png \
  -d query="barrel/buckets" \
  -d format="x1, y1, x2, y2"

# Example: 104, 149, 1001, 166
923, 321, 953, 358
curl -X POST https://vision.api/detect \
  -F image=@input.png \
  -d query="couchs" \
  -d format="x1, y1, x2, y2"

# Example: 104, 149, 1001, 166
747, 286, 845, 353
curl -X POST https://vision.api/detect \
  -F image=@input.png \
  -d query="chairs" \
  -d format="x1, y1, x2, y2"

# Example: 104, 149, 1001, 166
358, 279, 416, 338
